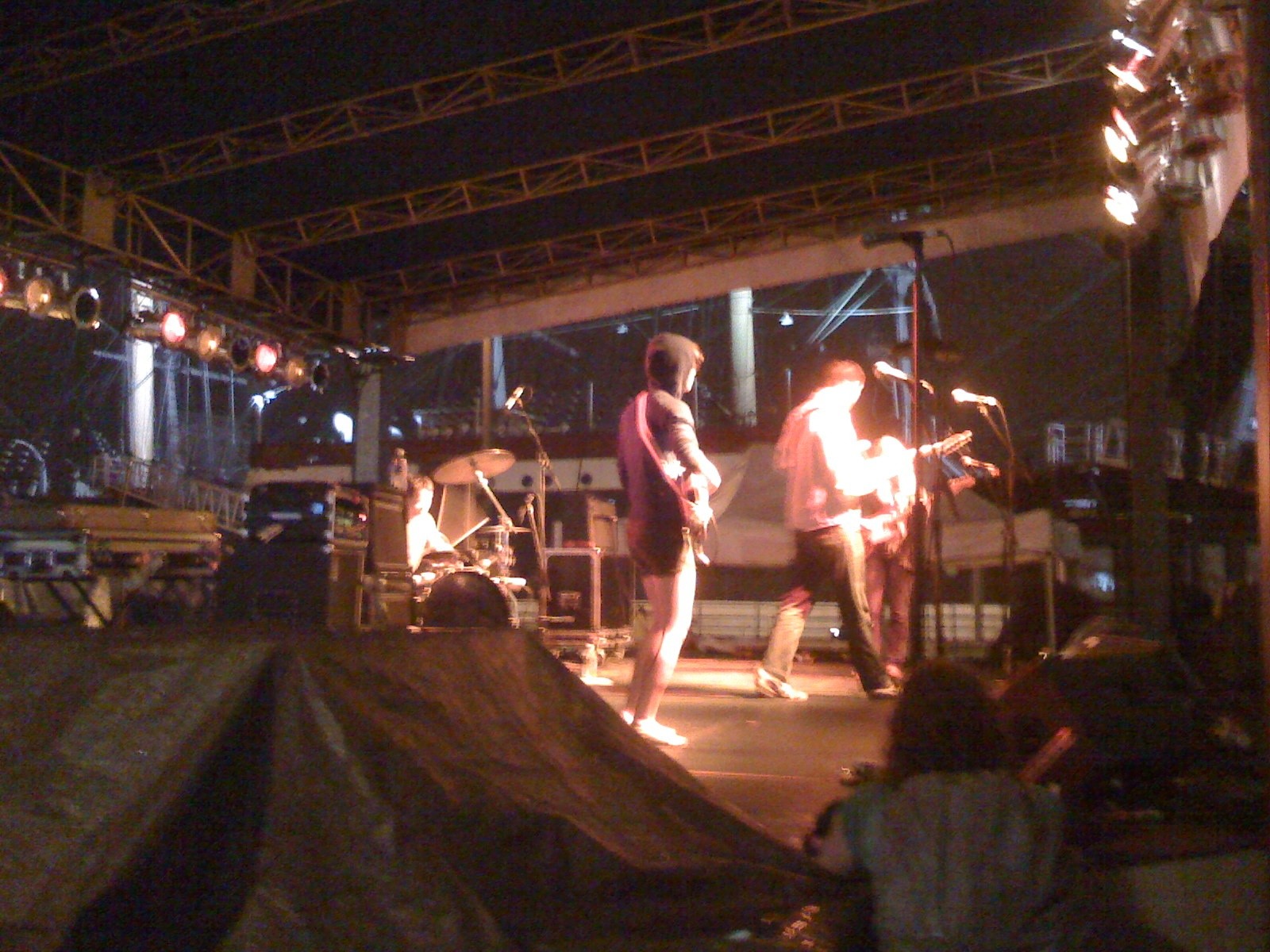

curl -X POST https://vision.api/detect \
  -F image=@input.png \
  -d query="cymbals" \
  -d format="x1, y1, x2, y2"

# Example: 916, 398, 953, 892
423, 548, 494, 565
431, 447, 518, 486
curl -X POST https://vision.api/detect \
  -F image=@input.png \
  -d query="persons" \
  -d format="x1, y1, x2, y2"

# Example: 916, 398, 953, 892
796, 659, 1098, 952
616, 332, 721, 747
751, 358, 933, 702
403, 474, 454, 577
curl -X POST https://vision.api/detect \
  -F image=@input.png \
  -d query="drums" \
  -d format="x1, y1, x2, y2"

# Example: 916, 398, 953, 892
424, 568, 520, 627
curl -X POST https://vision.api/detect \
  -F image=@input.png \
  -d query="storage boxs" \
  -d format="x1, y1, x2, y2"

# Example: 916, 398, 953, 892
538, 546, 635, 664
0, 523, 91, 579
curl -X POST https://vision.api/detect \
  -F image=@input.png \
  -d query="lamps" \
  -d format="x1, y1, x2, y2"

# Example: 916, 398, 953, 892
179, 324, 227, 364
256, 351, 310, 390
209, 334, 255, 374
0, 257, 58, 321
244, 335, 284, 379
779, 311, 794, 326
1096, 0, 1245, 261
44, 270, 104, 332
615, 321, 630, 335
130, 308, 189, 352
303, 358, 330, 393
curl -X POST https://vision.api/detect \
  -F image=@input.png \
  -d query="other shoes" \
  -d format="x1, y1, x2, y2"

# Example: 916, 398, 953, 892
866, 683, 901, 698
630, 723, 685, 747
620, 711, 675, 734
754, 668, 809, 700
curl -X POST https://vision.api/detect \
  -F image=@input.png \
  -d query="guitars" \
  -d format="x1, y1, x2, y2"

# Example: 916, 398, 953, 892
859, 464, 1002, 560
670, 441, 718, 545
